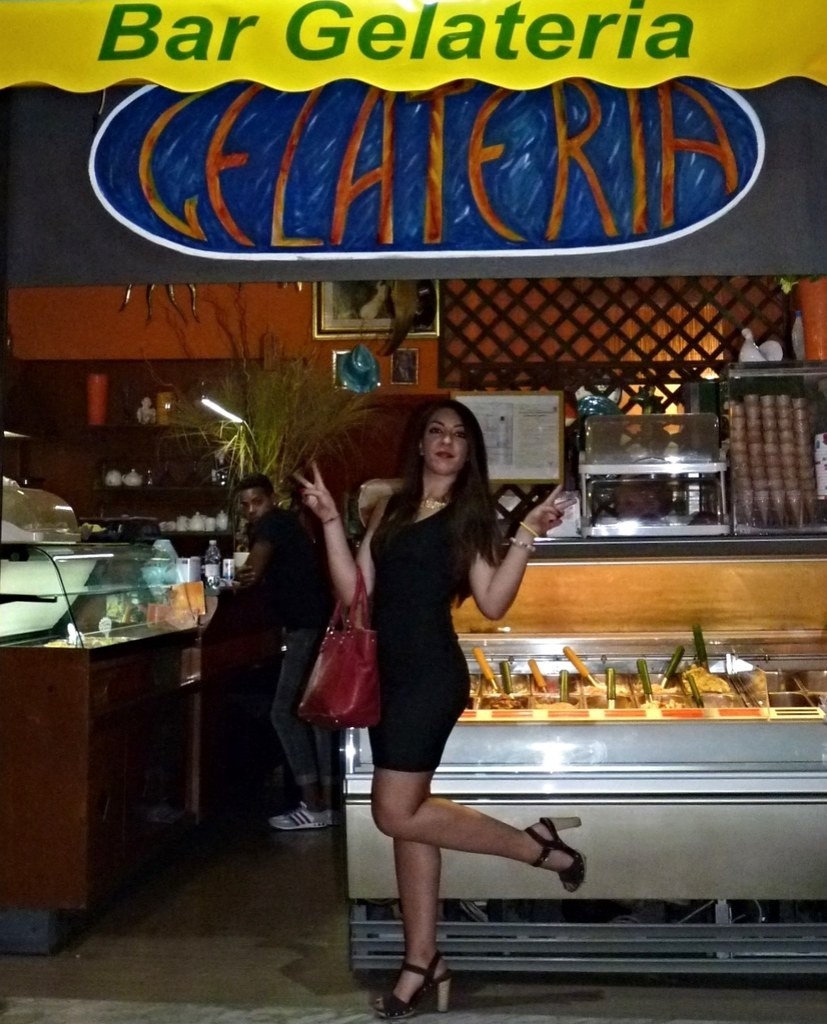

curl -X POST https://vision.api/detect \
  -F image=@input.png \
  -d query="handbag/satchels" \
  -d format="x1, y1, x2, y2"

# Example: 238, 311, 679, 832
299, 563, 382, 730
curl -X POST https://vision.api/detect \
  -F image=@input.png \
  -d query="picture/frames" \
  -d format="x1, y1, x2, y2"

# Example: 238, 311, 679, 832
331, 349, 352, 390
313, 279, 441, 340
389, 348, 419, 385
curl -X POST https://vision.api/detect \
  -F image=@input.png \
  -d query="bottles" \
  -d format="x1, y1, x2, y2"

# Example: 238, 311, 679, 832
792, 311, 806, 360
157, 382, 176, 424
204, 540, 219, 591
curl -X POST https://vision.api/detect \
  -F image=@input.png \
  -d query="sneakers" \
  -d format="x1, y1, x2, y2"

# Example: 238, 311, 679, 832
268, 803, 341, 830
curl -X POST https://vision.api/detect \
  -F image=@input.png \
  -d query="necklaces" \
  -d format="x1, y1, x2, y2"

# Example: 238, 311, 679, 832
421, 493, 449, 511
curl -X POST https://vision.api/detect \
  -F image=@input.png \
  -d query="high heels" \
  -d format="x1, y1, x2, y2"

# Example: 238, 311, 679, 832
525, 817, 585, 893
374, 951, 452, 1018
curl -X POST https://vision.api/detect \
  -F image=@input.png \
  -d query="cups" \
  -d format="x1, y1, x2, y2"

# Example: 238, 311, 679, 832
203, 517, 216, 532
84, 373, 110, 426
177, 516, 187, 532
222, 559, 235, 580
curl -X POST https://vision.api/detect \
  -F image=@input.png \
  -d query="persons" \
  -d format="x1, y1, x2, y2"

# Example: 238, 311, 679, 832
232, 470, 343, 831
290, 397, 590, 1018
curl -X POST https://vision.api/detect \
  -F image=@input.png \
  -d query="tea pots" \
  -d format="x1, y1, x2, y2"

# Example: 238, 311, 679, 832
183, 512, 205, 531
216, 510, 228, 531
122, 470, 144, 486
105, 470, 122, 486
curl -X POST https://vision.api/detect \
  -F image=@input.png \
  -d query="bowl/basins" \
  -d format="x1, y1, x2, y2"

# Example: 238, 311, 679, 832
234, 552, 250, 569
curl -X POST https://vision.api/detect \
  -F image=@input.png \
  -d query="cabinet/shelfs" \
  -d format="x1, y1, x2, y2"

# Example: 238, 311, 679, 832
50, 422, 241, 555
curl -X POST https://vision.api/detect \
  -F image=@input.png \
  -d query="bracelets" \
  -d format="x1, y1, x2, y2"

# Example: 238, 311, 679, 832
509, 536, 537, 553
321, 511, 343, 524
519, 520, 540, 541
